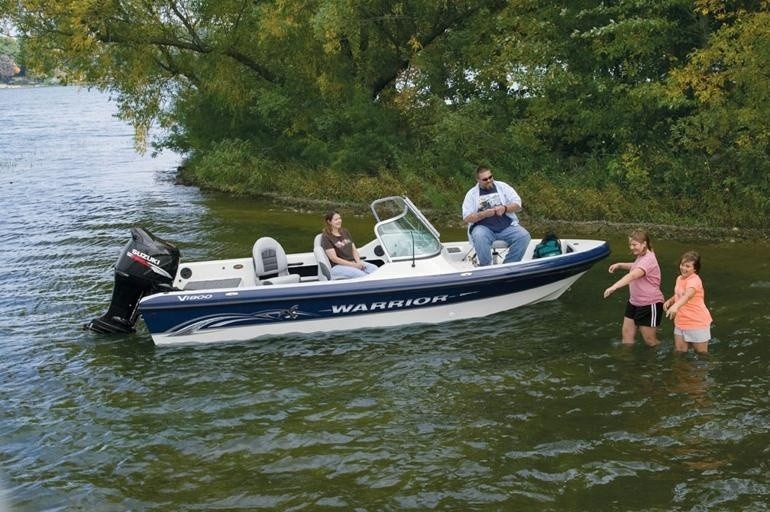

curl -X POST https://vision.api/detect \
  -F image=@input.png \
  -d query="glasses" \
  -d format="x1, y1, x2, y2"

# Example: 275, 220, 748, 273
482, 175, 493, 181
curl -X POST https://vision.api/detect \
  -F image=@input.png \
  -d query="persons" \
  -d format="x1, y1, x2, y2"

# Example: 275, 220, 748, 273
320, 209, 379, 280
661, 250, 714, 353
462, 164, 532, 267
603, 227, 665, 347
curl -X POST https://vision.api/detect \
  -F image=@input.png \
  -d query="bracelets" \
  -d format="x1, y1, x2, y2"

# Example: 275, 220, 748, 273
504, 205, 508, 213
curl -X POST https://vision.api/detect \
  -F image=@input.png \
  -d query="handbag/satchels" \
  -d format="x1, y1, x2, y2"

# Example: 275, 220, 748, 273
532, 233, 562, 259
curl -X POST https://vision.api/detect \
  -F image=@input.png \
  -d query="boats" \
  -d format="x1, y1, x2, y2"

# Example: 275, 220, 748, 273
79, 195, 612, 347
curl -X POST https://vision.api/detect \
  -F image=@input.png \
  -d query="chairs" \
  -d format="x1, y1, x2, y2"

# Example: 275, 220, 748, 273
313, 233, 349, 281
251, 236, 301, 286
461, 238, 508, 267
